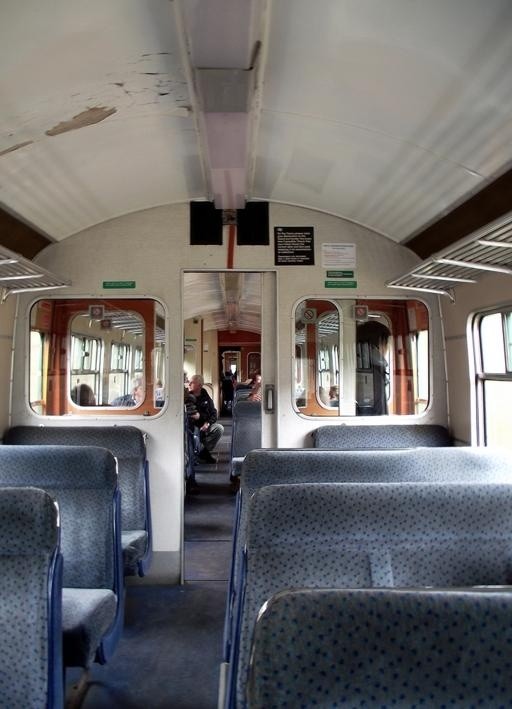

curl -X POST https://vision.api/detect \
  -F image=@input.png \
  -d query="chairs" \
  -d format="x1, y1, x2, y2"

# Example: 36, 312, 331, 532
229, 379, 265, 478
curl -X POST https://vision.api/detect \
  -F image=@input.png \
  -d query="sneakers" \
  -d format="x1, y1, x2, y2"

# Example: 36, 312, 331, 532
199, 447, 216, 463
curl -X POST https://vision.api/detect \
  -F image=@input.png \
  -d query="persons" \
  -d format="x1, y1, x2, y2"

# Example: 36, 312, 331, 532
354, 320, 394, 414
246, 383, 262, 402
329, 385, 339, 401
68, 371, 237, 496
246, 373, 261, 389
